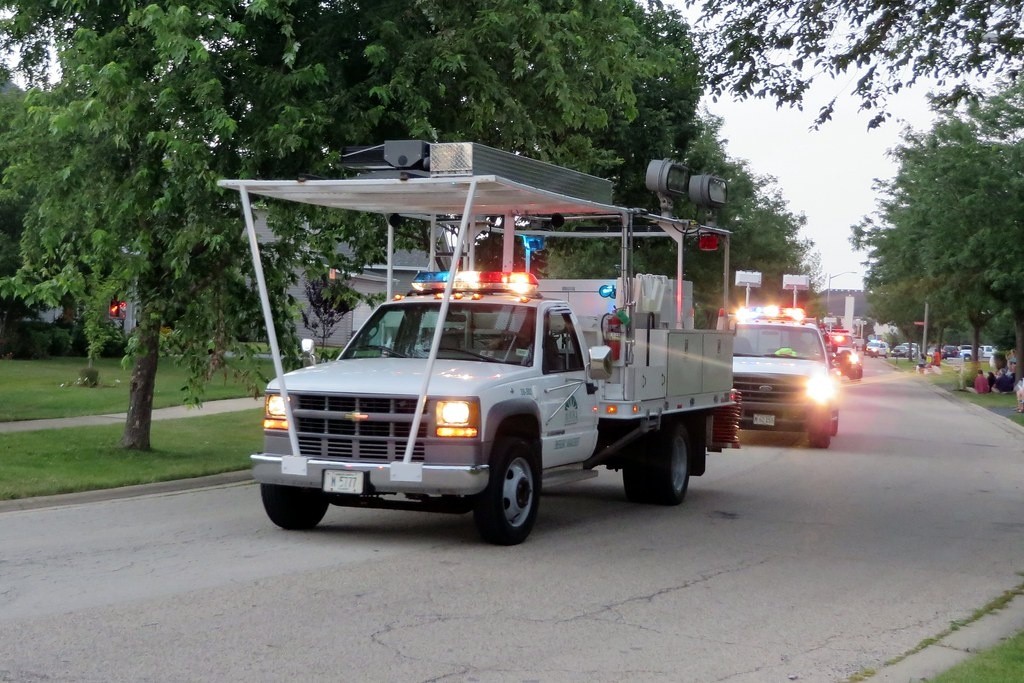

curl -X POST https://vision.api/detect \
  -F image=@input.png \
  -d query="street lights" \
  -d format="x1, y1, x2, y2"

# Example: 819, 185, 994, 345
828, 271, 857, 314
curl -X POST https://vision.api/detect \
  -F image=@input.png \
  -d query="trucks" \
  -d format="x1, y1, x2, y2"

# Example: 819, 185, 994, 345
217, 137, 746, 546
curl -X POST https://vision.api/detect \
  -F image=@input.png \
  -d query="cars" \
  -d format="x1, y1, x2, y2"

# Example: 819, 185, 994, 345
824, 327, 889, 379
891, 345, 908, 357
718, 306, 842, 452
943, 346, 960, 357
903, 343, 920, 358
927, 348, 948, 361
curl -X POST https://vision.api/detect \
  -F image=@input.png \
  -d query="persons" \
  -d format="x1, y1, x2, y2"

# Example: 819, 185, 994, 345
918, 354, 925, 367
934, 349, 940, 366
974, 370, 990, 393
987, 371, 996, 388
941, 348, 948, 359
991, 368, 1013, 394
1016, 388, 1024, 413
489, 316, 533, 350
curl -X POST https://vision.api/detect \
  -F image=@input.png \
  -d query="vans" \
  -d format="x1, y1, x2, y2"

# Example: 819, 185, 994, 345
955, 345, 997, 362
